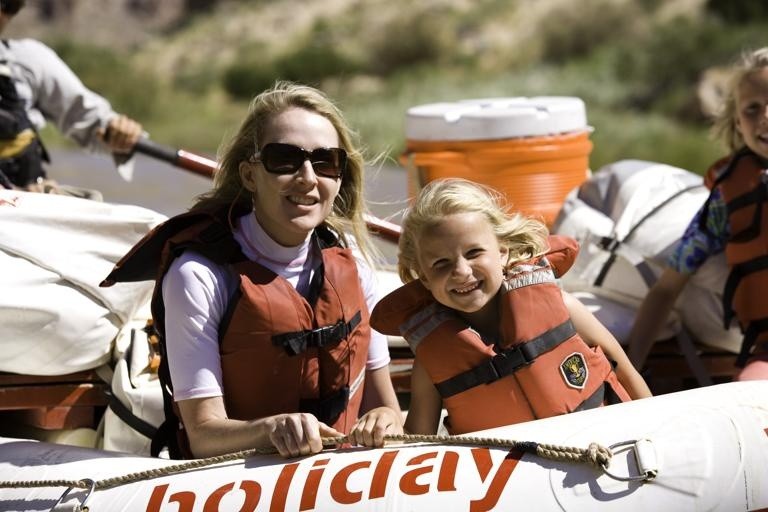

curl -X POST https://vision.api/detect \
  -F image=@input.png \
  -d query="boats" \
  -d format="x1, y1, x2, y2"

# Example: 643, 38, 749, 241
0, 267, 768, 512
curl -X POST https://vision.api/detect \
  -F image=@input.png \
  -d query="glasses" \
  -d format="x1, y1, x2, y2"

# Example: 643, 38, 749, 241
249, 142, 348, 179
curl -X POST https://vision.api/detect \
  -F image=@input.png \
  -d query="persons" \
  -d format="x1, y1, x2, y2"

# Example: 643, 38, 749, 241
0, 0, 147, 194
97, 77, 398, 458
370, 176, 649, 435
627, 46, 768, 379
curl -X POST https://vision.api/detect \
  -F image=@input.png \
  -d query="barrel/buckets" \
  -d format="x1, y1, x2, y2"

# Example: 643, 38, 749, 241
397, 95, 596, 238
